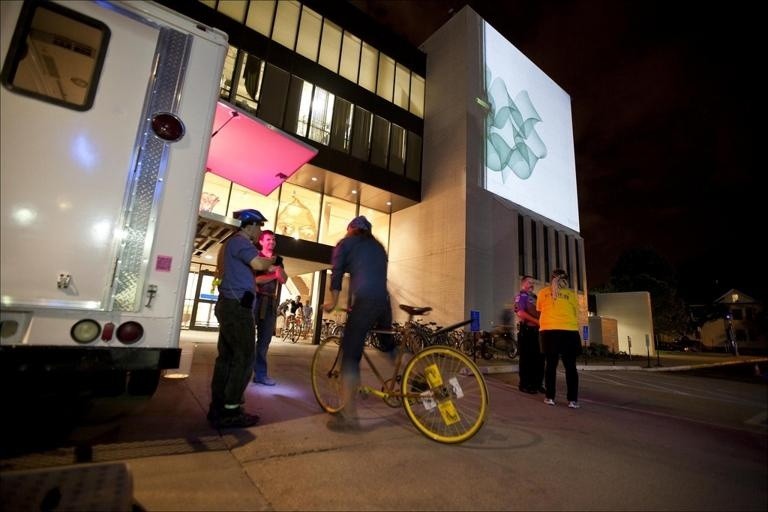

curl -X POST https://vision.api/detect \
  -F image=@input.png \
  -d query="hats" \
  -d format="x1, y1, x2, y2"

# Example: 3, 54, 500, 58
551, 269, 568, 280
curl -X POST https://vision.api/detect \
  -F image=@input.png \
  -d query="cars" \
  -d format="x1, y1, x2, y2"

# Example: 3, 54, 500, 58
664, 339, 680, 351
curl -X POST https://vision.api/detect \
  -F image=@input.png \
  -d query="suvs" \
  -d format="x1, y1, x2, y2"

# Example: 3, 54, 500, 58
671, 339, 705, 353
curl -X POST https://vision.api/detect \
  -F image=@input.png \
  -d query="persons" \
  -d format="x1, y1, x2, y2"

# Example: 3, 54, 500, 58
203, 208, 277, 431
246, 230, 287, 386
321, 214, 426, 434
274, 292, 313, 344
511, 276, 545, 395
535, 268, 582, 409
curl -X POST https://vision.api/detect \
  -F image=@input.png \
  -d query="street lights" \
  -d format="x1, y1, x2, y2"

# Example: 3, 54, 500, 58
726, 312, 736, 355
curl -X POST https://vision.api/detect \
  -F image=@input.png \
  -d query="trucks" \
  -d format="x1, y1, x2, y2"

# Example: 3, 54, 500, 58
4, 3, 319, 464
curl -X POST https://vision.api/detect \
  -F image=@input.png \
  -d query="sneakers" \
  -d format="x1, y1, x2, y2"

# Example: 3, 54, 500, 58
539, 384, 545, 393
568, 401, 580, 408
411, 374, 431, 393
208, 409, 244, 418
326, 413, 360, 433
519, 385, 538, 394
544, 397, 555, 405
254, 374, 276, 386
212, 412, 260, 428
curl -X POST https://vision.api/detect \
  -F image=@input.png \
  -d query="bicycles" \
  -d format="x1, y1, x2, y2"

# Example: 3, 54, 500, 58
309, 302, 491, 448
281, 311, 521, 364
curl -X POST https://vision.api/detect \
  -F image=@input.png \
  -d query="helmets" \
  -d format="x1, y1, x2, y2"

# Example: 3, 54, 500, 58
240, 208, 267, 223
